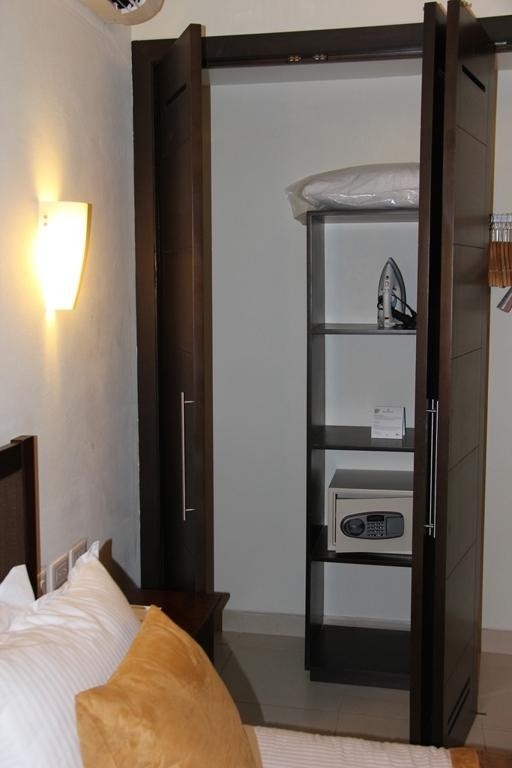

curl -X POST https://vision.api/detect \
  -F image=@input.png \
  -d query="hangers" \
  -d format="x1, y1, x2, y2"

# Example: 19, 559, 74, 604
482, 211, 511, 292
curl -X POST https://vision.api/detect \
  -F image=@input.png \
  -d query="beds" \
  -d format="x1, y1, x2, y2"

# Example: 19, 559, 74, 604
0, 432, 512, 767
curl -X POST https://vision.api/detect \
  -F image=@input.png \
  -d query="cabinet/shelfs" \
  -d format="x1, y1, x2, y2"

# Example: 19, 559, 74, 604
303, 208, 421, 691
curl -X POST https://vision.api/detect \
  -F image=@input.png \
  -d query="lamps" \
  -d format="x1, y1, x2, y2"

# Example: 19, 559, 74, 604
35, 196, 93, 321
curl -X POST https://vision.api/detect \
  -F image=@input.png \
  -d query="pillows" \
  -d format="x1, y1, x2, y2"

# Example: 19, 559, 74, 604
0, 540, 143, 766
74, 603, 254, 768
0, 562, 39, 646
298, 160, 419, 212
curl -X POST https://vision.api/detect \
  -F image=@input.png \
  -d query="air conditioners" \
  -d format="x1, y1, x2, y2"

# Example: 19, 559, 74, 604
84, 0, 163, 28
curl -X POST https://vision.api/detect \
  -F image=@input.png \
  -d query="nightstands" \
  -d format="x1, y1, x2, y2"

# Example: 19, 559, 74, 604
119, 582, 231, 665
